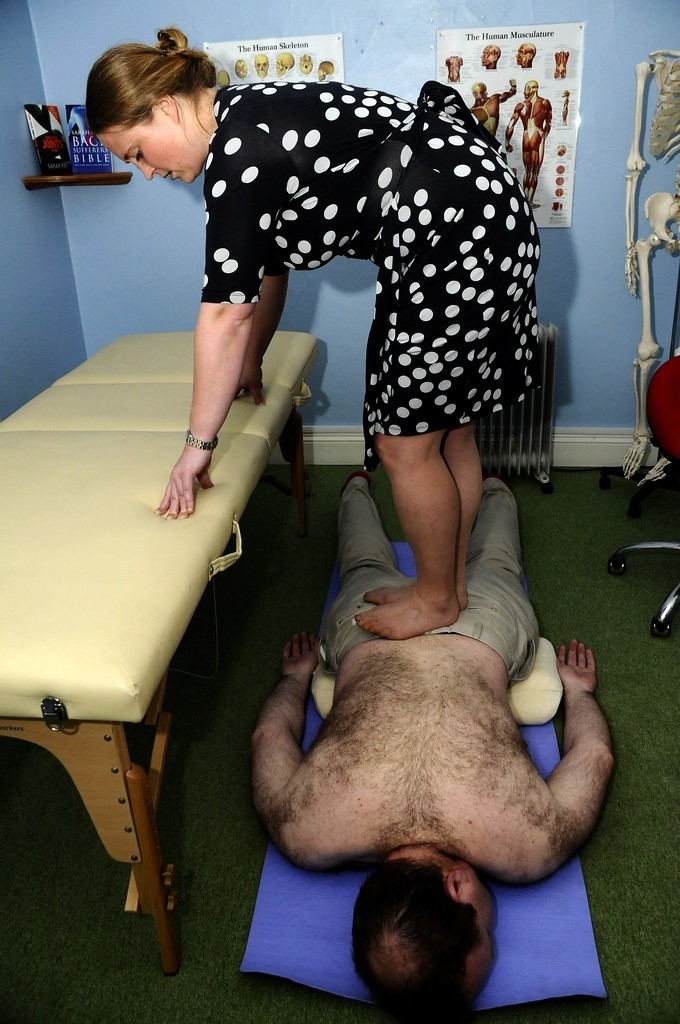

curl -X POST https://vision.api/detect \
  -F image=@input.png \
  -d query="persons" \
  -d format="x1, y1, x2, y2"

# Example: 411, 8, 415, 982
86, 27, 540, 640
250, 470, 613, 1024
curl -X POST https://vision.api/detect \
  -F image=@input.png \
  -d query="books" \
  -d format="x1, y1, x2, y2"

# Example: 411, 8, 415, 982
24, 104, 113, 177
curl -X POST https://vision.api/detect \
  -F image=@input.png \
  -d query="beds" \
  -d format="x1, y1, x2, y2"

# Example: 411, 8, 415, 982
0, 332, 323, 974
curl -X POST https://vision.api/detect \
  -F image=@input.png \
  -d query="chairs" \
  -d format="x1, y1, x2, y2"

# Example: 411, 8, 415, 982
606, 355, 680, 639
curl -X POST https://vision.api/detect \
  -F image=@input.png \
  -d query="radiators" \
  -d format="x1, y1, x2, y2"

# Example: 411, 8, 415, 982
472, 321, 561, 495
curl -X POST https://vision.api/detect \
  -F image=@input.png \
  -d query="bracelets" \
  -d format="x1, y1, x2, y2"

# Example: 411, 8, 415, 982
185, 429, 218, 450
